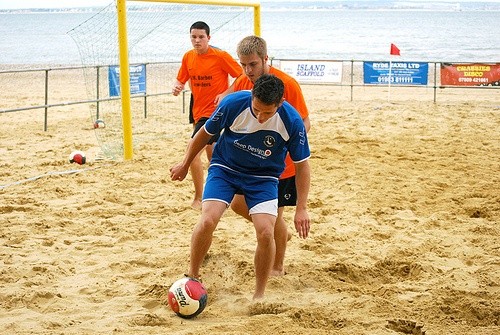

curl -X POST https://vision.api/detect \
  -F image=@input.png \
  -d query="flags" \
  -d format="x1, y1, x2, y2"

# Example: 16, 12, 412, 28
390, 43, 400, 56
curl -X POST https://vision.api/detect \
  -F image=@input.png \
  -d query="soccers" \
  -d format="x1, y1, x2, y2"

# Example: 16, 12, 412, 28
94, 119, 105, 129
69, 151, 86, 165
168, 277, 209, 319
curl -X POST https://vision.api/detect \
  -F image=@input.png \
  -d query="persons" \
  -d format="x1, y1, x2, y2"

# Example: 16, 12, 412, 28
229, 34, 311, 277
170, 73, 311, 305
170, 21, 247, 209
